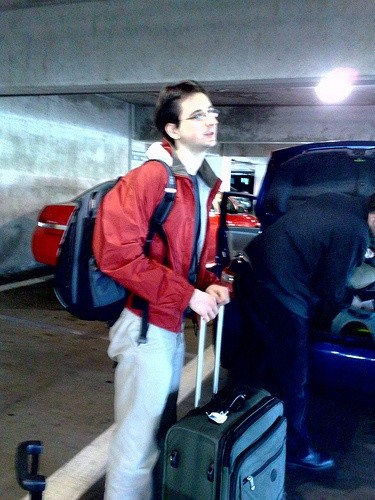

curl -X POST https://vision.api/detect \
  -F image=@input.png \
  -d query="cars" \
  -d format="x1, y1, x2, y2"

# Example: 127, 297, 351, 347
33, 177, 259, 269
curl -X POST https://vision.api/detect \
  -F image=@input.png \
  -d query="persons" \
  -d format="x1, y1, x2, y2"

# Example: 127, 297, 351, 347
243, 193, 375, 472
92, 79, 235, 500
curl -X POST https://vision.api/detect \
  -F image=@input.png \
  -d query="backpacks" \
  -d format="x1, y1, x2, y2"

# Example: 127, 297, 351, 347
52, 159, 175, 322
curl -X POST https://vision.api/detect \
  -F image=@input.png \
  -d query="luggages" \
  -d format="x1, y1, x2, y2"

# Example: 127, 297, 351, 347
160, 301, 289, 500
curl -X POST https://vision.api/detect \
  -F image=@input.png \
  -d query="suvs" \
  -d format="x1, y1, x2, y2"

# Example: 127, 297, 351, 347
254, 141, 375, 400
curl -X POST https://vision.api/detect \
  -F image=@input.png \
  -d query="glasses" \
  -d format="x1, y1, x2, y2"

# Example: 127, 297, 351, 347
175, 108, 220, 123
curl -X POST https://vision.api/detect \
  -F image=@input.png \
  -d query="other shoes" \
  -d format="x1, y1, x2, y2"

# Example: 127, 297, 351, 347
290, 449, 335, 470
